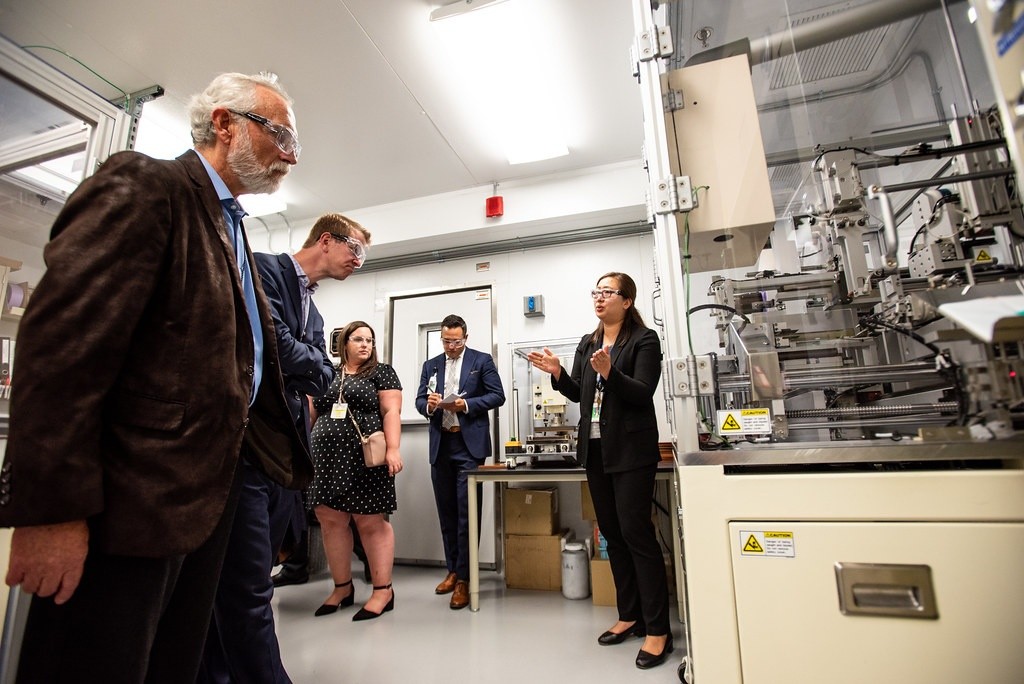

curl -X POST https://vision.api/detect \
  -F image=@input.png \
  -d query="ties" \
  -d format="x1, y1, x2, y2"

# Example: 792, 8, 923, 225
442, 359, 456, 430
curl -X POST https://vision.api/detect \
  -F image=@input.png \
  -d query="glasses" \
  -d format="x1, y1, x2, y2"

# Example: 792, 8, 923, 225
227, 107, 297, 159
330, 232, 369, 269
591, 290, 622, 298
440, 338, 465, 346
349, 336, 376, 348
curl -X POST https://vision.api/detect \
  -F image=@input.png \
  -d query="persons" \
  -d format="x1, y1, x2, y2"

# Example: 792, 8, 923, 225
0, 72, 302, 684
526, 272, 675, 670
214, 213, 403, 684
415, 314, 506, 611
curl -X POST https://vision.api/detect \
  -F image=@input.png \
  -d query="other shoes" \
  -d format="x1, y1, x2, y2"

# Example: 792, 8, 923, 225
365, 562, 372, 582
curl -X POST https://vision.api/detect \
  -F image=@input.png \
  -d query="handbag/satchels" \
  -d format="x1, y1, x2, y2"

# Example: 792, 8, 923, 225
362, 431, 387, 468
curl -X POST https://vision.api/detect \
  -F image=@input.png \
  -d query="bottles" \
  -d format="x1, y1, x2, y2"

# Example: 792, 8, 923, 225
561, 542, 590, 600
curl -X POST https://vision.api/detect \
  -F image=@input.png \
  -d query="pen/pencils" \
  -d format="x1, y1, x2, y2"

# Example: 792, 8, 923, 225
426, 385, 434, 393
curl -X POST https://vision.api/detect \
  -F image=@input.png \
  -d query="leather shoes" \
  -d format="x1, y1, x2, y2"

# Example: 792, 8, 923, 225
450, 580, 470, 609
435, 572, 458, 594
271, 565, 309, 587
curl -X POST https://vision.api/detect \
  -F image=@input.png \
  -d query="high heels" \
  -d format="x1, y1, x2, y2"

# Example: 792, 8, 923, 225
598, 618, 647, 646
636, 633, 673, 669
315, 578, 355, 616
353, 582, 394, 620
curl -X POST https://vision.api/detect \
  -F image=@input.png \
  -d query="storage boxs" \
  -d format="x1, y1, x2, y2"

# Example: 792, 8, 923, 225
504, 528, 569, 591
503, 487, 559, 536
581, 481, 618, 607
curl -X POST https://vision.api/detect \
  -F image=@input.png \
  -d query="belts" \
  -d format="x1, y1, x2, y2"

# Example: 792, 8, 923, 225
442, 425, 460, 432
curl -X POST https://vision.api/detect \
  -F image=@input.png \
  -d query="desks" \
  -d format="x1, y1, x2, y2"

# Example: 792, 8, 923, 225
460, 461, 684, 623
